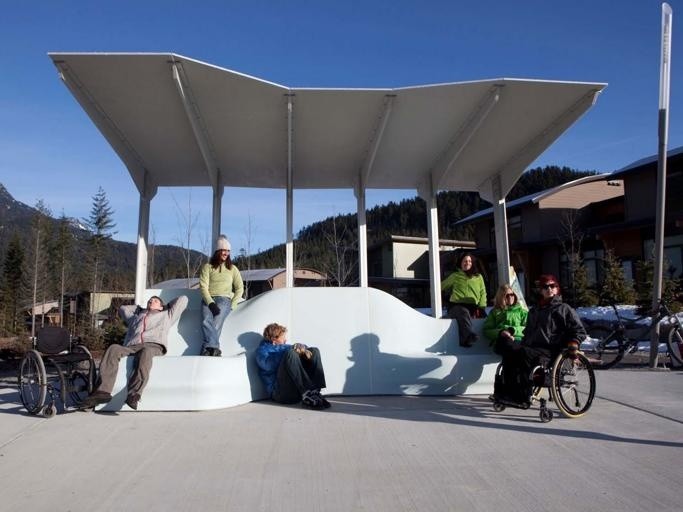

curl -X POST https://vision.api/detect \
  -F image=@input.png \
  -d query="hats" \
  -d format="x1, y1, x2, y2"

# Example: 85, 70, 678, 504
264, 323, 285, 340
215, 235, 231, 252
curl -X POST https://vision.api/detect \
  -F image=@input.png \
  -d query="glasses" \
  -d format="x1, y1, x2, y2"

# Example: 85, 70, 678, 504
542, 283, 558, 289
505, 293, 514, 298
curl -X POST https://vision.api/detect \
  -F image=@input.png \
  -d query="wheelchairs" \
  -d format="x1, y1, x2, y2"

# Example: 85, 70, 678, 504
489, 350, 595, 423
18, 325, 97, 414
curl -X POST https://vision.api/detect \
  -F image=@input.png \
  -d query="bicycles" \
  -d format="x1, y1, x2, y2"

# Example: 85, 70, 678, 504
578, 295, 682, 370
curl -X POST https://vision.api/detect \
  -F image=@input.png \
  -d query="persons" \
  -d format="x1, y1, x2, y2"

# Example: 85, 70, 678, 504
199, 235, 244, 356
82, 296, 190, 408
496, 275, 587, 405
256, 324, 330, 409
442, 252, 486, 348
483, 285, 529, 355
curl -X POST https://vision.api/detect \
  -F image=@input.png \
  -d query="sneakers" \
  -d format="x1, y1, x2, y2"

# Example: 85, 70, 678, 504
200, 347, 222, 356
125, 393, 140, 409
302, 391, 331, 409
84, 391, 112, 406
460, 334, 480, 347
531, 364, 550, 381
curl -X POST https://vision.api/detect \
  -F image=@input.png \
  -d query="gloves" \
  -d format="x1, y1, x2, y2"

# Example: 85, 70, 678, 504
568, 338, 581, 359
207, 302, 220, 316
476, 307, 485, 317
